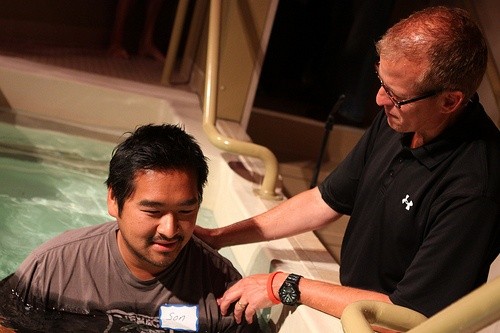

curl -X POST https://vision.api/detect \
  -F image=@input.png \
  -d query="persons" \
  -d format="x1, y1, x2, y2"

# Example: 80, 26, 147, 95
193, 5, 500, 331
8, 122, 260, 333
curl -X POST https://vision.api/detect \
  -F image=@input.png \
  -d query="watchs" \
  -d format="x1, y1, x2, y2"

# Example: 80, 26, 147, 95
278, 273, 302, 306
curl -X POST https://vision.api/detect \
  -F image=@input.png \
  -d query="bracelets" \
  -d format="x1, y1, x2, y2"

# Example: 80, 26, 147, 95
267, 271, 283, 304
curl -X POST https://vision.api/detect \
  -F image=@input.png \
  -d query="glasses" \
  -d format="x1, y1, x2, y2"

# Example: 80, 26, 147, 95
375, 70, 448, 109
